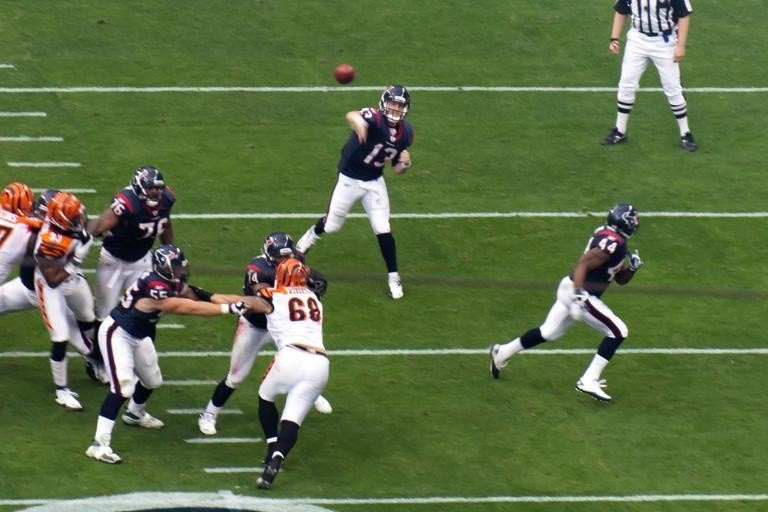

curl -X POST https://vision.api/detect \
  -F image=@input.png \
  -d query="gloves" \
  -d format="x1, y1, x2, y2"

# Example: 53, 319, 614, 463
627, 247, 644, 271
72, 233, 94, 264
221, 300, 251, 316
570, 294, 588, 321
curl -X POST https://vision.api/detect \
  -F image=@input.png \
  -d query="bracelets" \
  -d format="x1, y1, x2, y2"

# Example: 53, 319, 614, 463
609, 38, 619, 42
401, 160, 413, 169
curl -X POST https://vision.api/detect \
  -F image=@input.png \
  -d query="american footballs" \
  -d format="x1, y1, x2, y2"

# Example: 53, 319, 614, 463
335, 64, 354, 85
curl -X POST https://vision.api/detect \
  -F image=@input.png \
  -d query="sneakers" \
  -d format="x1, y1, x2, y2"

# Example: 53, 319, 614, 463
84, 438, 125, 468
489, 343, 508, 381
196, 411, 220, 437
680, 134, 699, 153
385, 272, 407, 302
85, 359, 111, 389
294, 225, 322, 256
599, 130, 629, 146
313, 392, 335, 417
260, 456, 284, 472
120, 406, 167, 431
255, 459, 282, 490
573, 378, 614, 405
52, 387, 86, 412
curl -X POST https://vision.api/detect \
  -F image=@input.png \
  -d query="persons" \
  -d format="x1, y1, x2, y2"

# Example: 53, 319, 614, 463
489, 202, 642, 403
182, 257, 330, 490
183, 231, 333, 436
600, 0, 699, 151
3, 166, 248, 462
296, 83, 414, 299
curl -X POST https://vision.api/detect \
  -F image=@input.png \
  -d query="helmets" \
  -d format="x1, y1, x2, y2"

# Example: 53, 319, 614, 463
377, 82, 413, 125
33, 188, 59, 220
150, 241, 192, 285
262, 230, 297, 268
272, 256, 312, 289
1, 181, 35, 218
129, 163, 168, 209
606, 201, 640, 234
48, 191, 87, 233
